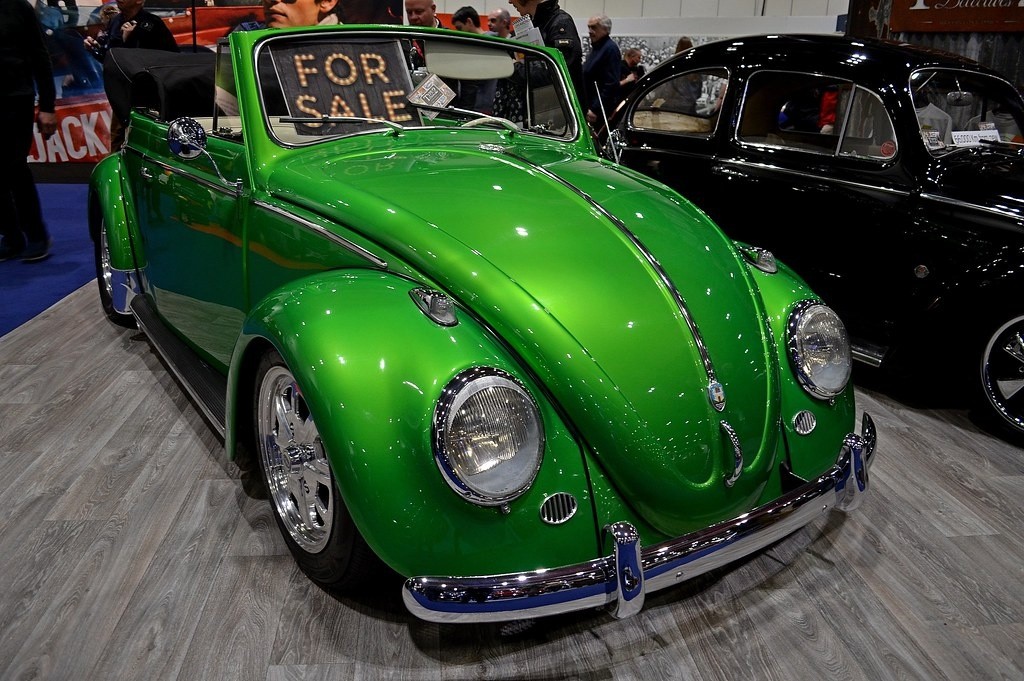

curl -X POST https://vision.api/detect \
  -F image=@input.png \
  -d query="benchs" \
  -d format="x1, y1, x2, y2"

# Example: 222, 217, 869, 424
196, 116, 319, 144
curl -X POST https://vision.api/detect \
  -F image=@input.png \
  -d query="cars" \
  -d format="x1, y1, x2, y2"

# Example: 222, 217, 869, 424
89, 22, 876, 627
593, 31, 1024, 449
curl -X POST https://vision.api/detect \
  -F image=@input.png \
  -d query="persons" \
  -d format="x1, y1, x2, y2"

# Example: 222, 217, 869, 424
0, 0, 56, 260
36, 0, 347, 92
674, 37, 702, 99
404, 0, 646, 130
82, 0, 177, 153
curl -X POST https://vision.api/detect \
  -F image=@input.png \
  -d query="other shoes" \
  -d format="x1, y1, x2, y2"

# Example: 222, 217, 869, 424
0, 248, 49, 261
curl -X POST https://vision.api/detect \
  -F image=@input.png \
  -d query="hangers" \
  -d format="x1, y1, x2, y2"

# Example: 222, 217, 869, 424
913, 89, 929, 108
